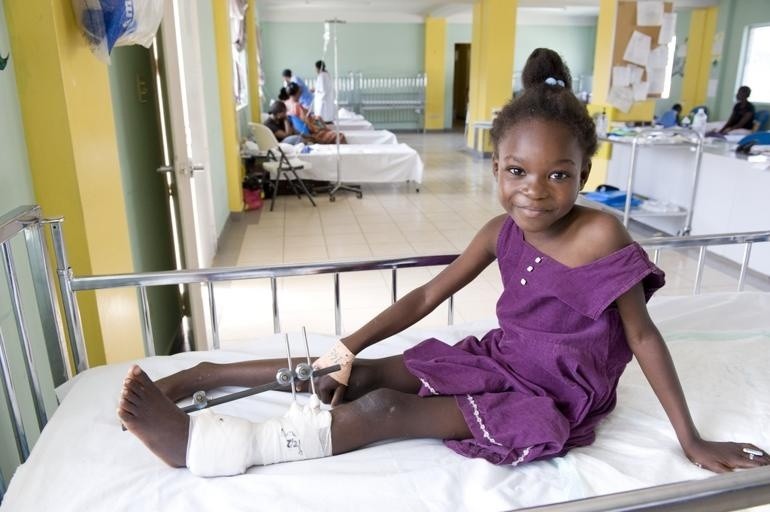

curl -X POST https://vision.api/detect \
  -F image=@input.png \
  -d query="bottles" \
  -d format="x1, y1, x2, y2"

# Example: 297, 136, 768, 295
692, 108, 707, 140
596, 110, 608, 139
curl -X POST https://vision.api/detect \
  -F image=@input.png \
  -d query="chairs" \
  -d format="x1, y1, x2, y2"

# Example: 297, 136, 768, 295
751, 110, 769, 132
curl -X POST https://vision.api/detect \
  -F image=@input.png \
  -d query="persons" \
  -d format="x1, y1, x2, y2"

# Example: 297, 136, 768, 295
115, 48, 770, 478
718, 86, 754, 134
658, 103, 683, 129
261, 59, 346, 144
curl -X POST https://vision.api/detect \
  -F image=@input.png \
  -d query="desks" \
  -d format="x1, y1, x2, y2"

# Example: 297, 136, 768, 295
471, 122, 493, 157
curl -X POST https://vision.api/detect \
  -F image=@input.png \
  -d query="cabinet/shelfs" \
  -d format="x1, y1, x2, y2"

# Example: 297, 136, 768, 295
584, 127, 706, 236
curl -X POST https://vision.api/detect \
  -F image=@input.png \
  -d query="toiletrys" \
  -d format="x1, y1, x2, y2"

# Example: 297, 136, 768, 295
595, 106, 609, 139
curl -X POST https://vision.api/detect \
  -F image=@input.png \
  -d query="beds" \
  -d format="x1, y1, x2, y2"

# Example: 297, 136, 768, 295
241, 100, 426, 211
0, 192, 768, 511
